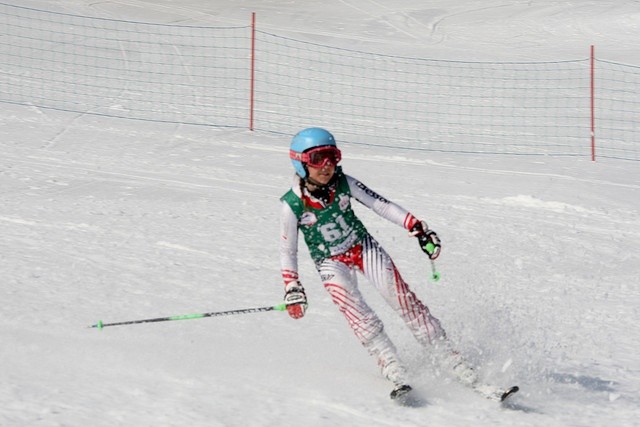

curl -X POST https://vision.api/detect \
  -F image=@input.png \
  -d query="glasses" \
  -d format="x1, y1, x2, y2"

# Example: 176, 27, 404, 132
290, 146, 341, 167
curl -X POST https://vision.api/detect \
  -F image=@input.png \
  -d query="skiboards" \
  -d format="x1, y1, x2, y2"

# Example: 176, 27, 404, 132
473, 380, 520, 403
390, 384, 414, 398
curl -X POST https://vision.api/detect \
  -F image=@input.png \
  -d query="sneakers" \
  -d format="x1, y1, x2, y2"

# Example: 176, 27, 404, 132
442, 357, 477, 384
381, 358, 409, 386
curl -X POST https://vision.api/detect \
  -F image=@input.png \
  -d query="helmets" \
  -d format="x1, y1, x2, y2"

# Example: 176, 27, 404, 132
290, 128, 336, 179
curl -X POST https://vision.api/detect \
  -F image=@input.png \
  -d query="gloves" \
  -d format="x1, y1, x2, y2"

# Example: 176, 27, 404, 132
284, 280, 307, 319
409, 220, 440, 259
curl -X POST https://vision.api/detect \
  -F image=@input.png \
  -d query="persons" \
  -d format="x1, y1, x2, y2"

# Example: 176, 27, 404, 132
280, 126, 479, 387
